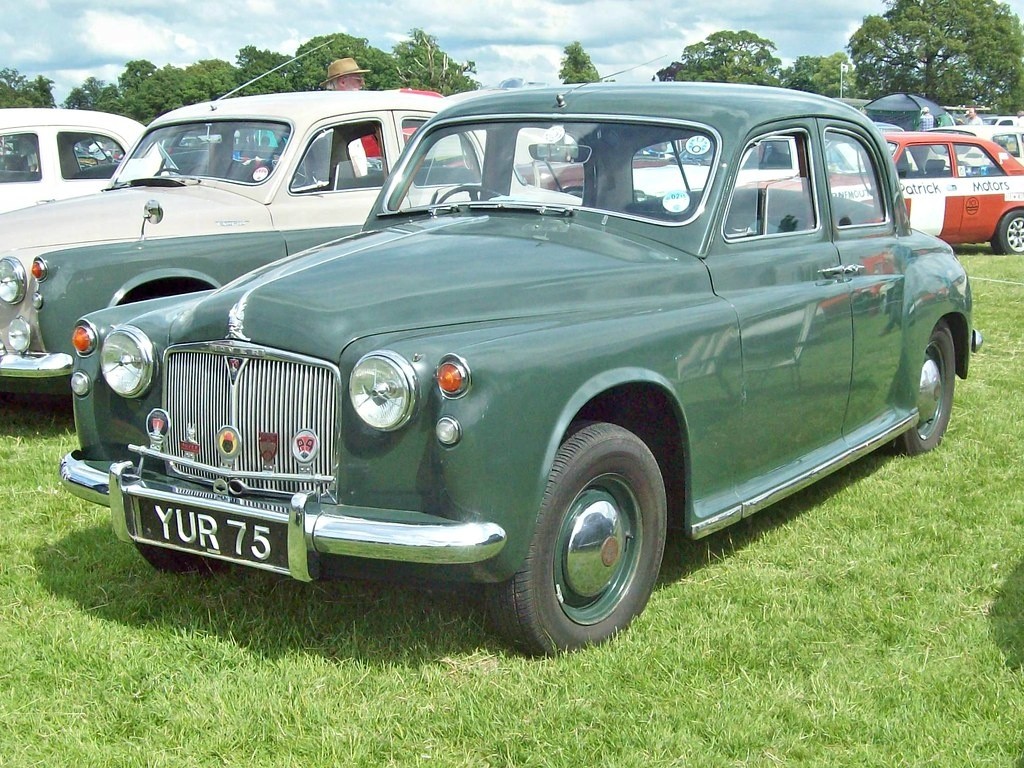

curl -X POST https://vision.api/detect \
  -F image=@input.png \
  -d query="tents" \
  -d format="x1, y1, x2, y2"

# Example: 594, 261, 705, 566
382, 88, 445, 144
831, 93, 953, 131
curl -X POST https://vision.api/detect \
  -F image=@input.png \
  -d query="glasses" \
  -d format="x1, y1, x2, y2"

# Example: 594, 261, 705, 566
344, 77, 365, 81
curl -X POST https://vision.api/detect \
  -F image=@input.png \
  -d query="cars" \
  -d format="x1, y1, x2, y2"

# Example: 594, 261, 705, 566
288, 86, 1024, 258
58, 81, 983, 661
0, 108, 184, 218
1, 87, 585, 407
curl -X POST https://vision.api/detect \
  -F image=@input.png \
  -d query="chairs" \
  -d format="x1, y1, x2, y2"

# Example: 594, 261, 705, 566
925, 159, 946, 177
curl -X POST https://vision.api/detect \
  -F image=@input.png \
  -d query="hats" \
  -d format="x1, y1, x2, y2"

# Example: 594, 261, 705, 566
319, 57, 371, 86
922, 107, 929, 113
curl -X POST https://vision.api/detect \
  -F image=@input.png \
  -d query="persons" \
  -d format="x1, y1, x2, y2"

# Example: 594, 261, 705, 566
304, 58, 370, 181
1016, 111, 1024, 126
964, 107, 983, 125
917, 107, 934, 131
